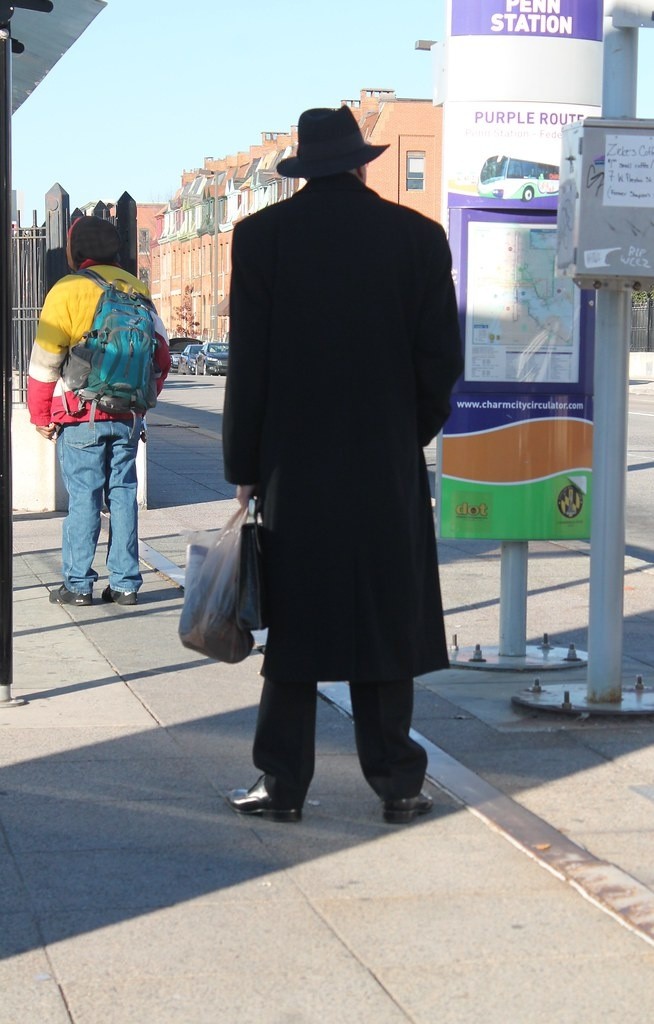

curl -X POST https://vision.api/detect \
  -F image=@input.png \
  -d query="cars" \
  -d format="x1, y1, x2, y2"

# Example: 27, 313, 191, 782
170, 354, 181, 369
178, 344, 203, 375
195, 342, 229, 376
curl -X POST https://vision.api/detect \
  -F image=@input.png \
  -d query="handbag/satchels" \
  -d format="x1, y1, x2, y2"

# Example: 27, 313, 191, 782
178, 497, 268, 664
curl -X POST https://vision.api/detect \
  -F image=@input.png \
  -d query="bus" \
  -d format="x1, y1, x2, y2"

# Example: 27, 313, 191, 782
474, 147, 561, 202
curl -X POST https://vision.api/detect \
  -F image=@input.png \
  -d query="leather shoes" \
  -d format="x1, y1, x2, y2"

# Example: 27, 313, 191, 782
226, 773, 300, 822
383, 792, 433, 823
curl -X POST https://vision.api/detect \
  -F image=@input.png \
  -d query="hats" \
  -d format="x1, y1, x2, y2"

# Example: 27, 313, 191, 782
277, 106, 391, 178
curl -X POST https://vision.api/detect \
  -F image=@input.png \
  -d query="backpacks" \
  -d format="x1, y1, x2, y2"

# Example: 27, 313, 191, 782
62, 268, 158, 414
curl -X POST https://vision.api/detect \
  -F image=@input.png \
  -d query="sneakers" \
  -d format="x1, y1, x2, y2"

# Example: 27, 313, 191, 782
50, 584, 93, 605
102, 585, 138, 604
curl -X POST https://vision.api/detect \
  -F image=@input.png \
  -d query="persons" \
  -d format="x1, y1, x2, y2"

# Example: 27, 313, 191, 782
26, 216, 170, 606
223, 105, 466, 824
